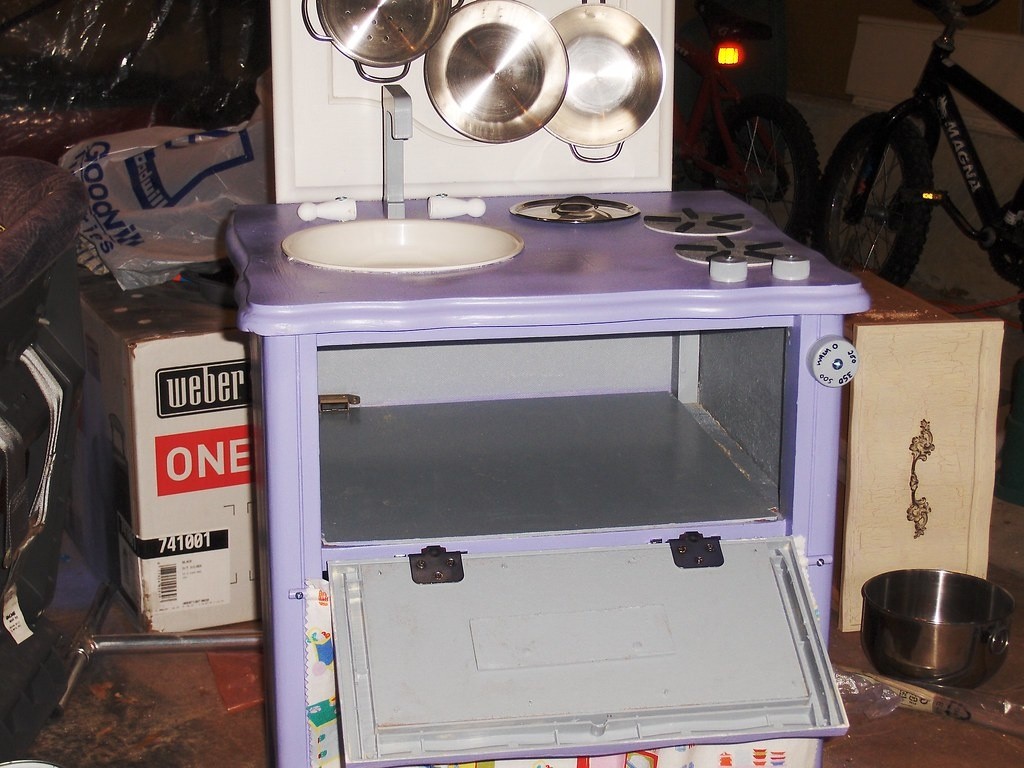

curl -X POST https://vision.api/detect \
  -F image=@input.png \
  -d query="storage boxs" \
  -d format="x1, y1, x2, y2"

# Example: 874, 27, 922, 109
78, 280, 264, 634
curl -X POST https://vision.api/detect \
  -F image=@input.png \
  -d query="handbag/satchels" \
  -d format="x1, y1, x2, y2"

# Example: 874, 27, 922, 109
51, 77, 275, 290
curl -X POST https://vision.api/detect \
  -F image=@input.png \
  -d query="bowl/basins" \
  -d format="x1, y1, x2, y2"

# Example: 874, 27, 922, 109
861, 568, 1016, 692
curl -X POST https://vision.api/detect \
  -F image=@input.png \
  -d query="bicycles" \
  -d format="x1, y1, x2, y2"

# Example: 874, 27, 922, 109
676, 1, 823, 251
810, 0, 1024, 327
